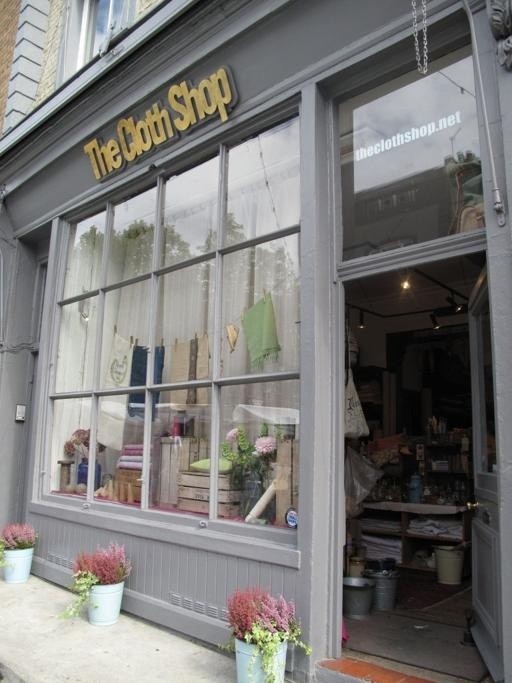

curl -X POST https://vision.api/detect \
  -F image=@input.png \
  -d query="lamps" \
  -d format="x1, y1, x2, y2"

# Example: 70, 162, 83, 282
430, 291, 463, 330
359, 308, 365, 328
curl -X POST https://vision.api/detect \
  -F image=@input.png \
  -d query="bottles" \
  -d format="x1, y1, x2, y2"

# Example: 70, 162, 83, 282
408, 471, 423, 503
447, 432, 470, 450
350, 556, 365, 578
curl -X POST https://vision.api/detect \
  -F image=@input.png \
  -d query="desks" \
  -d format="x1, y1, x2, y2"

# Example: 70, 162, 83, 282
153, 435, 208, 508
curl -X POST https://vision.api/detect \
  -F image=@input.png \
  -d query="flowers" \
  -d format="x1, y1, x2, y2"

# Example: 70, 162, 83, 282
221, 582, 314, 681
1, 520, 39, 568
65, 428, 107, 458
221, 420, 283, 491
49, 537, 133, 618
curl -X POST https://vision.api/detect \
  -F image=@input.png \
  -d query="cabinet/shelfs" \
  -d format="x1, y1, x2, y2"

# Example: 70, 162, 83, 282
354, 499, 469, 574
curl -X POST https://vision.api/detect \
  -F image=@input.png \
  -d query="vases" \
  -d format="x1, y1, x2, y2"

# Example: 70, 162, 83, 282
240, 478, 278, 522
5, 548, 36, 583
78, 457, 102, 491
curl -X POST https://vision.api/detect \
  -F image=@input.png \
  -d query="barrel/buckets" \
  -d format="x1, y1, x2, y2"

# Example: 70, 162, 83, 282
362, 568, 401, 610
343, 576, 377, 616
435, 545, 466, 585
2, 546, 35, 583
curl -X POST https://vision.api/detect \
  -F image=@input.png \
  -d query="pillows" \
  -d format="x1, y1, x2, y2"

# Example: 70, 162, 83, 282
191, 456, 232, 474
